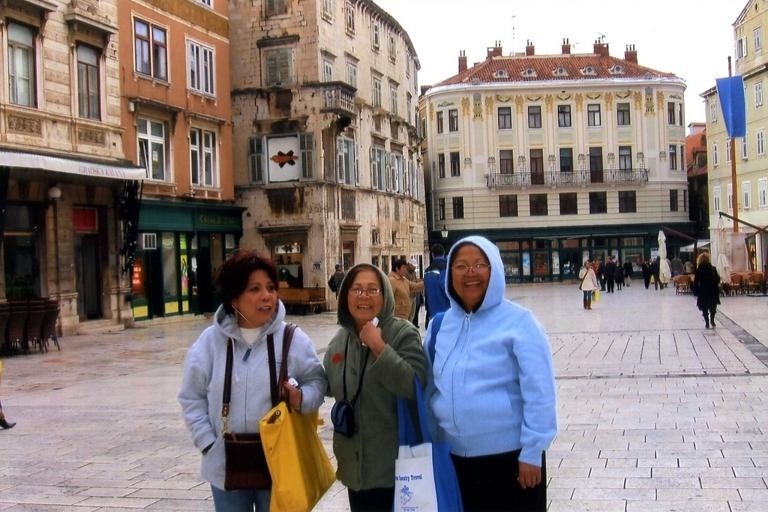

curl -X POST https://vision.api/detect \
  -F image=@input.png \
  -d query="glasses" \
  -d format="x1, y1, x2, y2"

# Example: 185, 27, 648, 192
348, 286, 384, 297
449, 262, 495, 275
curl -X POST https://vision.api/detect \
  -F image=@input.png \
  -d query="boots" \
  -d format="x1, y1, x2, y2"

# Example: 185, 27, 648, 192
703, 313, 717, 330
582, 298, 593, 310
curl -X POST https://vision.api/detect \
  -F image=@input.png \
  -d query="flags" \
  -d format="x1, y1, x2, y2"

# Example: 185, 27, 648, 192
715, 74, 746, 141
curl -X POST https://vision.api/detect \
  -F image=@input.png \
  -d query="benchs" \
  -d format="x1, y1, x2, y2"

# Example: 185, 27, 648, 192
276, 286, 327, 315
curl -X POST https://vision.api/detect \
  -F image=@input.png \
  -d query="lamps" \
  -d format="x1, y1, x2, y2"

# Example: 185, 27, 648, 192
423, 225, 449, 251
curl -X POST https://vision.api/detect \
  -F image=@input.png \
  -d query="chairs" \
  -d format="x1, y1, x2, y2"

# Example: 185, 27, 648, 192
1, 295, 62, 358
672, 268, 767, 298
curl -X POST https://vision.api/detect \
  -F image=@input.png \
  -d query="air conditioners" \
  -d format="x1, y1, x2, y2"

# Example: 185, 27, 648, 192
136, 231, 158, 253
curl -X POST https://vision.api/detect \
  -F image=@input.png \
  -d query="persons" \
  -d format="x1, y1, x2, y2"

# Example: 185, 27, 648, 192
691, 252, 722, 328
179, 249, 327, 511
0, 403, 16, 430
644, 257, 667, 289
327, 262, 343, 292
323, 262, 427, 512
387, 242, 448, 321
425, 234, 558, 512
576, 251, 633, 328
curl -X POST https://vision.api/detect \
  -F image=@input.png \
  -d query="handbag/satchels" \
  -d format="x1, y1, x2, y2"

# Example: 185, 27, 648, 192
327, 399, 364, 438
222, 421, 285, 491
391, 370, 463, 512
251, 398, 340, 512
593, 288, 602, 304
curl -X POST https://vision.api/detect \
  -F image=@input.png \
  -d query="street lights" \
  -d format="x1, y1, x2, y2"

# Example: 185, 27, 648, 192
50, 187, 66, 341
441, 223, 450, 255
